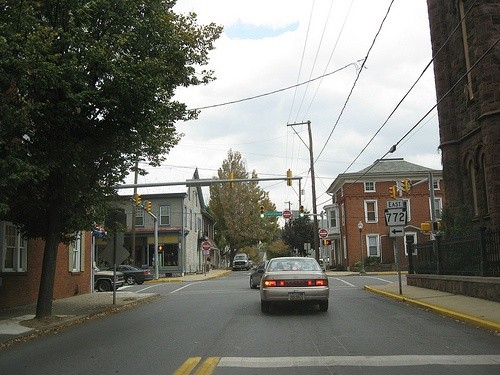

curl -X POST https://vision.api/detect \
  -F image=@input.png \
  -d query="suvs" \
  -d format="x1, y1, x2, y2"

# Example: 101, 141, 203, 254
93, 261, 126, 292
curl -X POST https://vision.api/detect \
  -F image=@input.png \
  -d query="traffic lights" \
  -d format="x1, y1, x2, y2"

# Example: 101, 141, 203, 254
136, 194, 141, 206
400, 178, 412, 195
146, 201, 152, 213
299, 205, 305, 218
388, 185, 397, 200
260, 206, 265, 218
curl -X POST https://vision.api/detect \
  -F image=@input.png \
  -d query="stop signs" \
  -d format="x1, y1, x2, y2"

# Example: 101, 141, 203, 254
318, 229, 328, 239
283, 211, 292, 218
201, 241, 211, 251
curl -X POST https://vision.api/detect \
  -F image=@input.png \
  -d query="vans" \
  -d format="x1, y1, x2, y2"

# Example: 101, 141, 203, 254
231, 252, 249, 271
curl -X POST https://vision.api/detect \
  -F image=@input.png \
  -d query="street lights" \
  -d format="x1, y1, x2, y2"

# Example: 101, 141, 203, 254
357, 221, 366, 276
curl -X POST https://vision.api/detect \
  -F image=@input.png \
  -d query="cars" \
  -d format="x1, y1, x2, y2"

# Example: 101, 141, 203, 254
257, 256, 330, 313
319, 259, 325, 271
105, 265, 156, 285
249, 259, 270, 289
